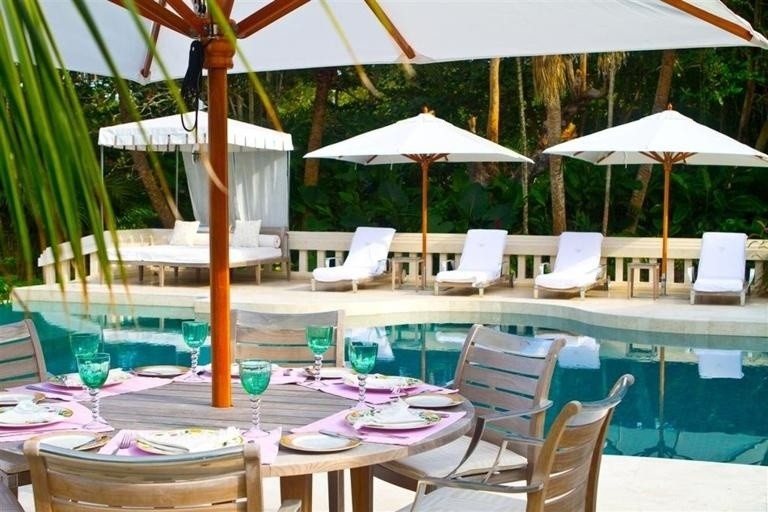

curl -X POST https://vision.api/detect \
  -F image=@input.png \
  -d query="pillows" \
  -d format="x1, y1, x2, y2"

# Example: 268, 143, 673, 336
232, 218, 261, 248
170, 217, 202, 248
194, 231, 280, 249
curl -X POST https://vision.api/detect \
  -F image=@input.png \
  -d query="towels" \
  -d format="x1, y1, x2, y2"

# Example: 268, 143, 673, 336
136, 423, 239, 456
0, 399, 65, 426
351, 398, 426, 431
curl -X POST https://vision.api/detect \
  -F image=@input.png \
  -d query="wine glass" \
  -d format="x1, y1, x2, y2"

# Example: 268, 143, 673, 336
345, 338, 379, 412
68, 331, 101, 401
78, 351, 111, 432
181, 318, 208, 384
237, 359, 272, 441
307, 323, 335, 390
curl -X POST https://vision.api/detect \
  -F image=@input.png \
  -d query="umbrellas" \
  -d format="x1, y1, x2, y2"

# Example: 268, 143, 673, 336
555, 341, 765, 470
300, 113, 536, 296
4, 2, 768, 413
539, 105, 768, 298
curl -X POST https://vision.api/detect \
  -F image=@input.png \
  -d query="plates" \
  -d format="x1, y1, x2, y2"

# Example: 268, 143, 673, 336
0, 404, 64, 428
46, 369, 124, 389
17, 431, 109, 451
297, 363, 345, 381
203, 361, 279, 376
279, 431, 362, 454
343, 372, 420, 394
405, 391, 463, 409
129, 365, 187, 377
134, 427, 245, 457
343, 404, 442, 432
2, 391, 45, 406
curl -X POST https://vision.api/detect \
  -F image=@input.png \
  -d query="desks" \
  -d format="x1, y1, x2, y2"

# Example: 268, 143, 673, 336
627, 343, 658, 365
625, 258, 660, 301
388, 255, 425, 292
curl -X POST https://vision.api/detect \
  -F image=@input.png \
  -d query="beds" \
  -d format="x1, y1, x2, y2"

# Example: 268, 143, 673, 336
99, 222, 293, 287
94, 313, 211, 356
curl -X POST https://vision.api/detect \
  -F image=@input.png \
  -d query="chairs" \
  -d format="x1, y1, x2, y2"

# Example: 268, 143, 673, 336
350, 322, 567, 512
344, 322, 397, 364
433, 227, 518, 297
223, 308, 345, 512
532, 330, 605, 375
534, 228, 611, 301
392, 372, 638, 511
433, 323, 502, 345
20, 438, 303, 512
310, 228, 400, 298
685, 229, 758, 308
0, 316, 48, 499
696, 349, 744, 380
